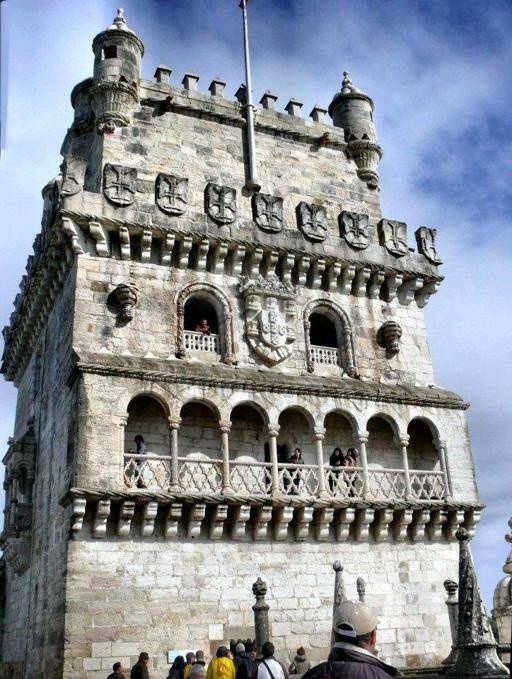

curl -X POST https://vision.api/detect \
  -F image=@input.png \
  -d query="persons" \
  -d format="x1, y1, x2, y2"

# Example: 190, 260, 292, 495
287, 448, 304, 495
130, 652, 150, 679
328, 448, 346, 495
343, 447, 360, 494
130, 435, 148, 490
108, 662, 126, 679
299, 598, 398, 679
195, 318, 211, 335
288, 647, 311, 675
166, 636, 288, 679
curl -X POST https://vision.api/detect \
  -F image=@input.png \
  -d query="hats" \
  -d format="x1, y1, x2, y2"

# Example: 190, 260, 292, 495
332, 598, 377, 637
137, 652, 149, 660
236, 643, 245, 652
297, 646, 306, 655
